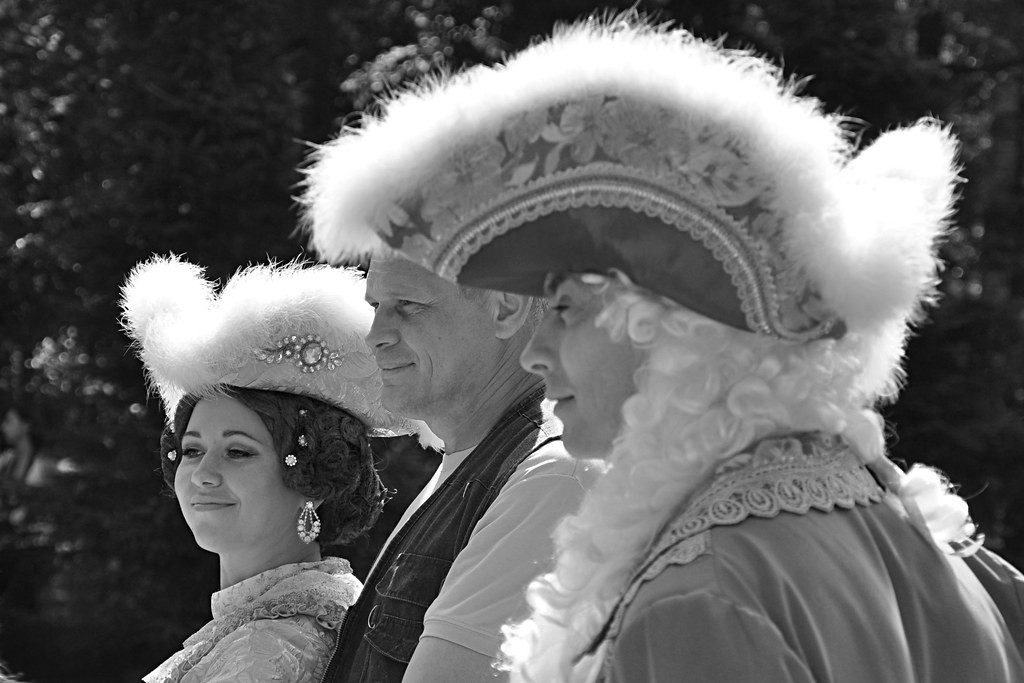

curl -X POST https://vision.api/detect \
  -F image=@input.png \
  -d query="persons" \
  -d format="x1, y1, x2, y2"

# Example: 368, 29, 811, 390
0, 404, 42, 482
118, 16, 1024, 683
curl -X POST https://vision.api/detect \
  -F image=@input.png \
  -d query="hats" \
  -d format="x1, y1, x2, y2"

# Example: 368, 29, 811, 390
296, 6, 962, 343
305, 63, 499, 258
120, 252, 443, 451
